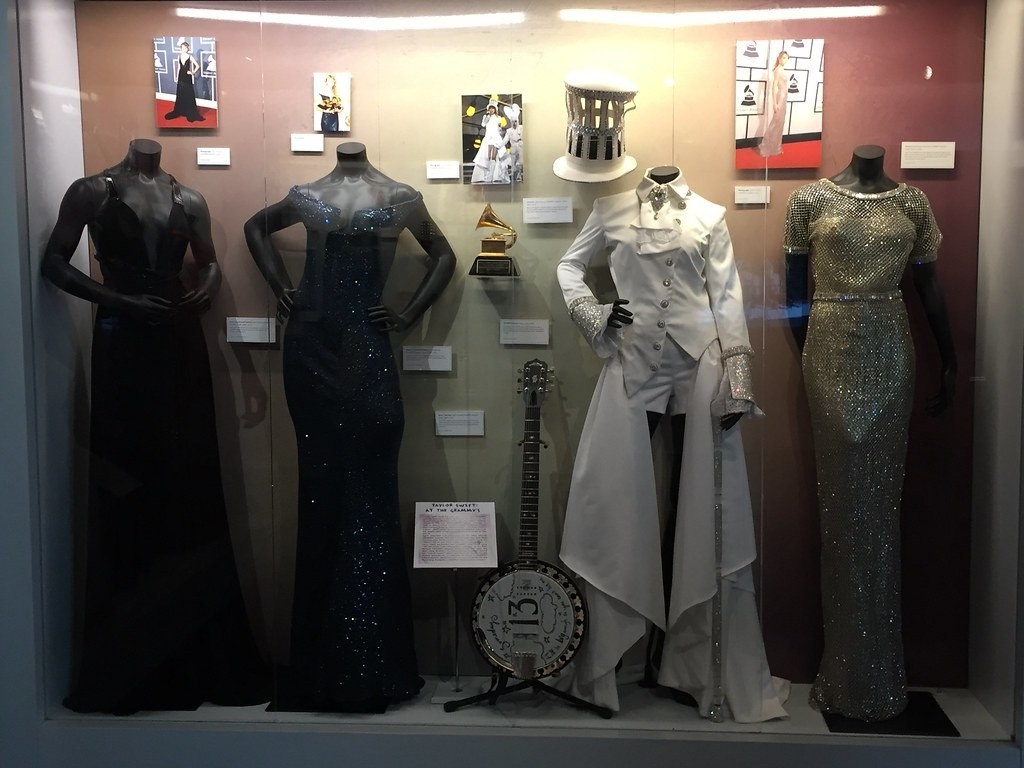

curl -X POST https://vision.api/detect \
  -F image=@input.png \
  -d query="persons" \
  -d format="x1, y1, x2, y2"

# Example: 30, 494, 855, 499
40, 140, 248, 713
470, 95, 525, 181
242, 141, 456, 706
757, 50, 790, 157
317, 74, 345, 131
558, 168, 792, 723
782, 144, 958, 722
164, 42, 207, 122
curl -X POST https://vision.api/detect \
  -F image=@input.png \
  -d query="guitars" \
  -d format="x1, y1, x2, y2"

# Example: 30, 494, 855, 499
467, 357, 591, 681
